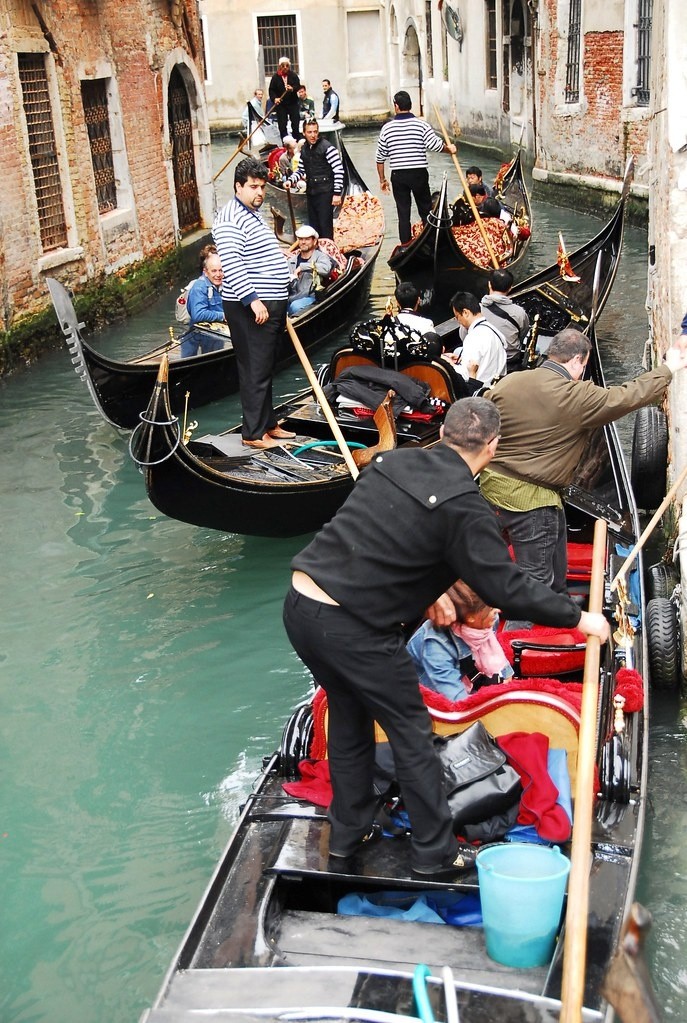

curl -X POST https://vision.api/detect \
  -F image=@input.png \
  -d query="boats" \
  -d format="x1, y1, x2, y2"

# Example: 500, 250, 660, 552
128, 156, 636, 537
44, 121, 388, 430
139, 420, 650, 1023
244, 99, 344, 211
387, 119, 536, 314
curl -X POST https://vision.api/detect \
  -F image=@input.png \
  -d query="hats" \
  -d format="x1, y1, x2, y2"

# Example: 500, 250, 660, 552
295, 225, 319, 239
297, 138, 306, 151
283, 135, 296, 145
279, 57, 291, 66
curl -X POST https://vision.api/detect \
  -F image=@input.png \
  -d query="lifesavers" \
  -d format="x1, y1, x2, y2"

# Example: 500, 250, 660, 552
629, 404, 680, 690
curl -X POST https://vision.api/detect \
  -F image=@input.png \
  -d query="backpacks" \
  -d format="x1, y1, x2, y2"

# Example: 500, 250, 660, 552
286, 269, 317, 303
175, 278, 213, 325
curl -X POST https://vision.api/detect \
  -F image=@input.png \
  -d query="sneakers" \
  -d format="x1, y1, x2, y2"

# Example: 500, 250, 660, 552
328, 819, 384, 858
411, 840, 478, 874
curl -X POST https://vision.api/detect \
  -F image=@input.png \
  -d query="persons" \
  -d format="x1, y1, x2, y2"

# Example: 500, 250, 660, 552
180, 223, 332, 360
404, 580, 518, 701
381, 268, 530, 398
241, 56, 342, 194
375, 91, 458, 247
214, 155, 301, 452
282, 397, 614, 875
450, 166, 520, 241
672, 313, 687, 359
282, 116, 345, 240
475, 328, 687, 629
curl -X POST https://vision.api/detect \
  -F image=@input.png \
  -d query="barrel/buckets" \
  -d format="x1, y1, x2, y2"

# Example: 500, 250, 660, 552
475, 842, 570, 968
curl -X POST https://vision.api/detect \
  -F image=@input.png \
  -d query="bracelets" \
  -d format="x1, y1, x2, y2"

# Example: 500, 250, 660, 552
380, 178, 386, 183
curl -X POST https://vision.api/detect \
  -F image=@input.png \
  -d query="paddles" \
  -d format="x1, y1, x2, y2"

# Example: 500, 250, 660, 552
432, 102, 500, 270
286, 182, 297, 242
558, 519, 607, 1023
286, 315, 360, 483
213, 90, 290, 180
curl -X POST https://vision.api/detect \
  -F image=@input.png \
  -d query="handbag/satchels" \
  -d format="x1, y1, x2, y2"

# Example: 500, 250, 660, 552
371, 719, 523, 842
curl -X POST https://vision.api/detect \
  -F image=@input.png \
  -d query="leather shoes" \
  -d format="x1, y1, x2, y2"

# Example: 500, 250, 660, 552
241, 433, 288, 451
266, 425, 297, 438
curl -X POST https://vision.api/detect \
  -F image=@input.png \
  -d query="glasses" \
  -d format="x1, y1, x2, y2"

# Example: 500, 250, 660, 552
280, 63, 290, 67
453, 314, 460, 321
